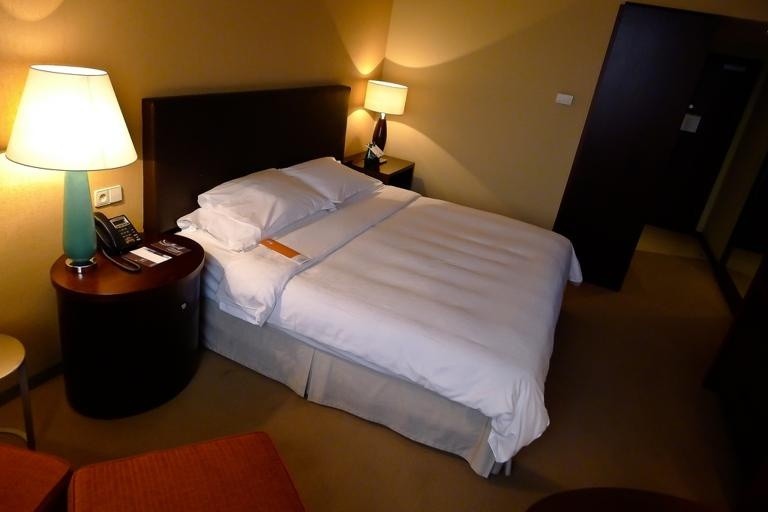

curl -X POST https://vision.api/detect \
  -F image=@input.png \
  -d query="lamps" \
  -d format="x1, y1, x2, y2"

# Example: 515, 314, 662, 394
364, 79, 409, 151
4, 64, 139, 271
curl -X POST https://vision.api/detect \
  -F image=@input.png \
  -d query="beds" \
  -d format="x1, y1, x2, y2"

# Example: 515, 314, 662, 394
141, 83, 574, 474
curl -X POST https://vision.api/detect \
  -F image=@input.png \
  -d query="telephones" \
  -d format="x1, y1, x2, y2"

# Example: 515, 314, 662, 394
93, 212, 143, 253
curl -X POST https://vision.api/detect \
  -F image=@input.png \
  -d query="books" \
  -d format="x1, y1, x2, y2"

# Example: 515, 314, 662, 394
122, 239, 192, 268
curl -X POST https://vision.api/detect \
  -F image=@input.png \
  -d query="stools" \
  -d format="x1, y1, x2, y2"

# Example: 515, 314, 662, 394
0, 333, 37, 451
66, 430, 308, 511
0, 441, 75, 511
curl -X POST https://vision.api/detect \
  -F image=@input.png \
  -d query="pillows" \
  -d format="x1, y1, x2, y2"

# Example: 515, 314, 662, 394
176, 156, 382, 254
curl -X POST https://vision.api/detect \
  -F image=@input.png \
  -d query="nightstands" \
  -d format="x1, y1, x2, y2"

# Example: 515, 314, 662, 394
50, 229, 205, 419
341, 151, 416, 190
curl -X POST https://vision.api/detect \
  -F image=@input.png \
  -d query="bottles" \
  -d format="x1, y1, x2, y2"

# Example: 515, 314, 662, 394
364, 142, 380, 166
